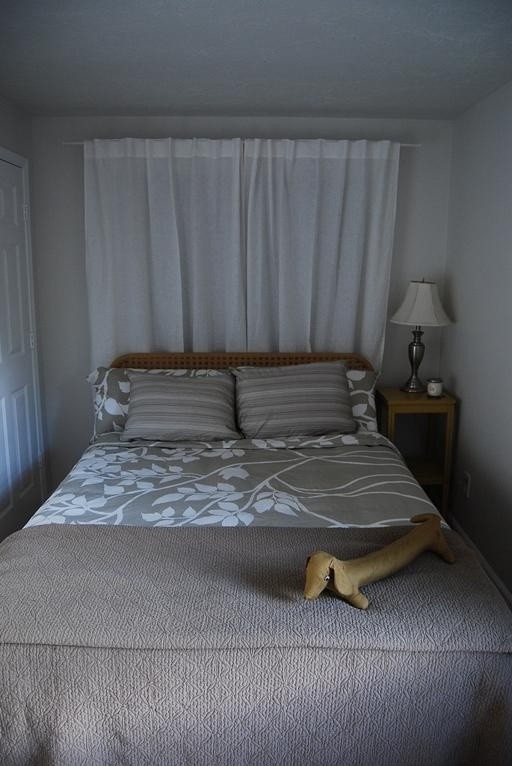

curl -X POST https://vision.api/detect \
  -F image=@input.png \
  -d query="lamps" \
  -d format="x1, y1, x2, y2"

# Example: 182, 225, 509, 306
389, 280, 454, 393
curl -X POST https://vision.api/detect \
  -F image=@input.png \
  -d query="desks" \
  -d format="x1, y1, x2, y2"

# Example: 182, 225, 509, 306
377, 388, 457, 520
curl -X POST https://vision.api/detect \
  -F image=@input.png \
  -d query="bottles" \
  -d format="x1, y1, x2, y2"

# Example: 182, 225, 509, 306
426, 377, 444, 398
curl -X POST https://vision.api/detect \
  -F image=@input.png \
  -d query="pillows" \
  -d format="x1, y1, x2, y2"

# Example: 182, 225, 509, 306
87, 360, 382, 443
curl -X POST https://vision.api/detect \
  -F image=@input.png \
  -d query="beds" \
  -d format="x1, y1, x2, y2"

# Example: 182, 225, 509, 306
1, 353, 511, 765
